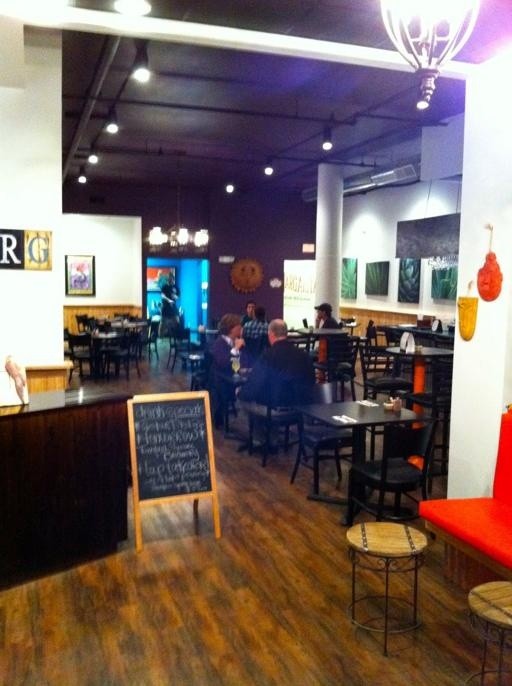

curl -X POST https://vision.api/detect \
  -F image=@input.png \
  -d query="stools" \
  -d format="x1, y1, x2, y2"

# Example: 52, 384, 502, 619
346, 523, 428, 654
464, 582, 512, 686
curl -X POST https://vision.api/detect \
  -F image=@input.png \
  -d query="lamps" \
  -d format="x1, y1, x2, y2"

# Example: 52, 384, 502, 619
264, 159, 274, 175
78, 165, 87, 184
146, 174, 209, 247
323, 125, 333, 150
106, 107, 119, 134
376, 1, 480, 103
133, 41, 151, 82
88, 139, 99, 165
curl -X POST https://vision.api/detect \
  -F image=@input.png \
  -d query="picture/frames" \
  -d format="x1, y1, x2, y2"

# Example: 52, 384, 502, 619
398, 258, 421, 303
342, 258, 358, 299
227, 257, 264, 294
147, 265, 177, 292
366, 262, 389, 296
65, 255, 95, 296
432, 266, 458, 300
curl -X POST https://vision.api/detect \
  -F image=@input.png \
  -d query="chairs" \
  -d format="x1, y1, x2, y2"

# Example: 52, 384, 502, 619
418, 405, 512, 582
410, 392, 452, 493
247, 377, 309, 467
289, 385, 353, 495
347, 413, 437, 526
67, 333, 94, 383
360, 340, 414, 433
103, 336, 134, 378
312, 336, 362, 400
75, 314, 97, 332
167, 327, 197, 372
136, 320, 161, 364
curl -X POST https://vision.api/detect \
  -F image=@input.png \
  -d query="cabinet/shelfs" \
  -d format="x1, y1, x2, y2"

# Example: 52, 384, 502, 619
0, 384, 134, 591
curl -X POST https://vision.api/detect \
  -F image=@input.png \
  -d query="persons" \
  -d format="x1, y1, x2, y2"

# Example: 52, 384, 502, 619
203, 314, 252, 426
234, 319, 315, 448
240, 300, 256, 325
158, 273, 182, 341
314, 304, 351, 382
243, 306, 270, 342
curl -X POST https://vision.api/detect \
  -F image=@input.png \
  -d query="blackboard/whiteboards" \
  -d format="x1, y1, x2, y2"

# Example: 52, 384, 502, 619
128, 392, 218, 505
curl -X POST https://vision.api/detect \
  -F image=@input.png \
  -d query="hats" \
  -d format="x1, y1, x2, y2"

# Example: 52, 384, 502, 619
314, 303, 332, 311
